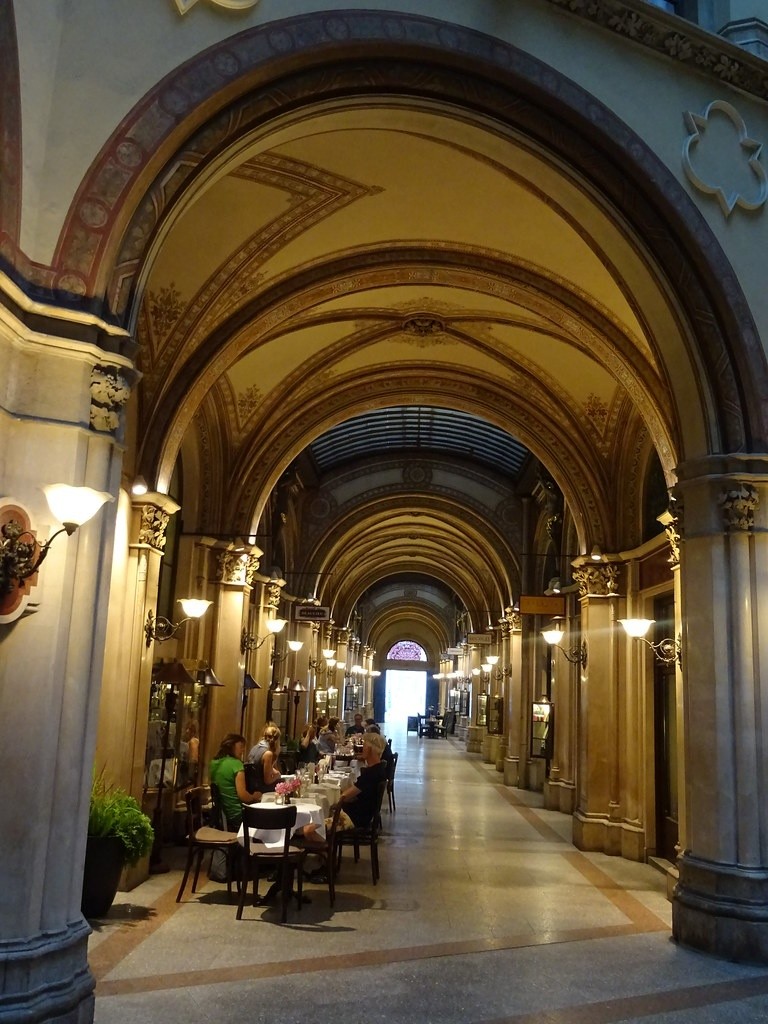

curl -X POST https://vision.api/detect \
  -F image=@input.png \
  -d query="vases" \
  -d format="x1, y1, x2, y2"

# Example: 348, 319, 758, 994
275, 792, 292, 803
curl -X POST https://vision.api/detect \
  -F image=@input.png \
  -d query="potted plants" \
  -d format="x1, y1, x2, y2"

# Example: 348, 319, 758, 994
80, 755, 158, 921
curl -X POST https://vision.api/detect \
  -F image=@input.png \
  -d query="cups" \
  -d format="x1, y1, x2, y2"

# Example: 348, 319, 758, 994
275, 795, 283, 804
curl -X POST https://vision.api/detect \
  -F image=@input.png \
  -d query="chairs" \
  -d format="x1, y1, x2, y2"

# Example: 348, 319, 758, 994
384, 753, 402, 815
243, 763, 266, 795
434, 715, 449, 740
417, 713, 432, 739
386, 738, 395, 751
231, 801, 307, 921
406, 715, 418, 736
290, 795, 347, 910
175, 784, 245, 906
328, 779, 389, 886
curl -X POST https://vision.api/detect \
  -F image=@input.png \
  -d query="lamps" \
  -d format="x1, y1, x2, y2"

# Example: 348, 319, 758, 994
268, 640, 308, 665
192, 666, 228, 696
289, 679, 309, 740
0, 476, 116, 605
129, 471, 151, 497
143, 598, 217, 649
432, 667, 489, 684
531, 693, 557, 714
239, 617, 290, 656
480, 663, 504, 682
539, 627, 587, 671
307, 648, 336, 670
330, 660, 384, 679
148, 659, 198, 876
613, 615, 684, 676
239, 672, 263, 736
485, 655, 512, 677
505, 540, 607, 615
315, 658, 337, 674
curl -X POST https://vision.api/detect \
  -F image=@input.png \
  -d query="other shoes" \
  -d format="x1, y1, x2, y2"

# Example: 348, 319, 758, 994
312, 865, 329, 875
311, 871, 330, 883
268, 870, 278, 881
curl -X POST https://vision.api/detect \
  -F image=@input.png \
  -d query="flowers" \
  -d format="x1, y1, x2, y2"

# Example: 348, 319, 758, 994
274, 779, 302, 794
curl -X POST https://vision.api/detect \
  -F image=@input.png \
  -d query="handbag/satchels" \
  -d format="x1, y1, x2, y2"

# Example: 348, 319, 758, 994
206, 846, 228, 881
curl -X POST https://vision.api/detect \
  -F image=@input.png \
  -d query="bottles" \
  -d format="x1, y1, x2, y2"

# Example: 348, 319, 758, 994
302, 775, 310, 799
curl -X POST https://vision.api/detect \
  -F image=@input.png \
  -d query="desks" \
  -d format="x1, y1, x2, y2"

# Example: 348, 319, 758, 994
243, 801, 323, 908
425, 718, 440, 738
260, 732, 373, 800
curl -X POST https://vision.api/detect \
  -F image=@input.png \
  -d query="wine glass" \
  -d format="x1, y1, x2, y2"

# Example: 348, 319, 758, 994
317, 764, 324, 786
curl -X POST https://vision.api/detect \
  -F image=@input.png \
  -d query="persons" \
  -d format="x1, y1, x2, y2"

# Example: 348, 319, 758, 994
300, 724, 324, 763
365, 720, 394, 780
249, 721, 285, 793
316, 716, 341, 753
208, 734, 262, 825
303, 733, 386, 884
345, 714, 364, 736
